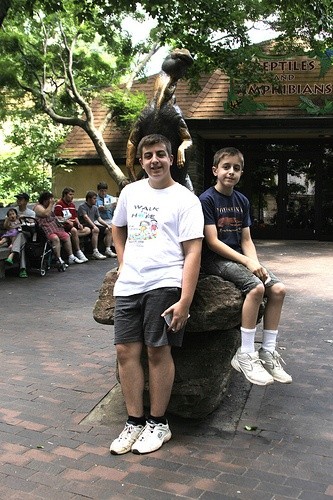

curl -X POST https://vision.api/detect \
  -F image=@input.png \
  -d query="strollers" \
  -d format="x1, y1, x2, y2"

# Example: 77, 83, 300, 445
17, 216, 67, 278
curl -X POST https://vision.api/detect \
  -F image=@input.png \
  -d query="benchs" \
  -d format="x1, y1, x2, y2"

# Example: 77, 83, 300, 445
0, 236, 104, 278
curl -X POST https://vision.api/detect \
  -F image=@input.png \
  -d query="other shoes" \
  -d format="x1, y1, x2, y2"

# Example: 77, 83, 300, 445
19, 271, 28, 278
5, 257, 13, 265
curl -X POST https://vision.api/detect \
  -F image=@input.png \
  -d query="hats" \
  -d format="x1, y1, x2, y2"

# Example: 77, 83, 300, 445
14, 192, 29, 198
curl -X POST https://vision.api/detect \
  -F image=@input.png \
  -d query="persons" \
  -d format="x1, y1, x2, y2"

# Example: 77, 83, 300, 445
110, 135, 205, 454
54, 188, 92, 262
200, 148, 292, 385
77, 182, 117, 259
0, 193, 36, 277
33, 192, 84, 267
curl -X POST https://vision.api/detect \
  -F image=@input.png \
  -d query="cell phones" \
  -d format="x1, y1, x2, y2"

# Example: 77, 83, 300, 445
164, 312, 176, 331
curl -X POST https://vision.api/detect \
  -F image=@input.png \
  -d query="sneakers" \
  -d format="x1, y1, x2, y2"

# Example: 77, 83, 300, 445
258, 347, 292, 384
56, 257, 69, 268
109, 418, 148, 455
92, 251, 107, 259
68, 257, 84, 265
77, 252, 88, 262
104, 250, 117, 258
230, 346, 274, 386
130, 414, 172, 455
